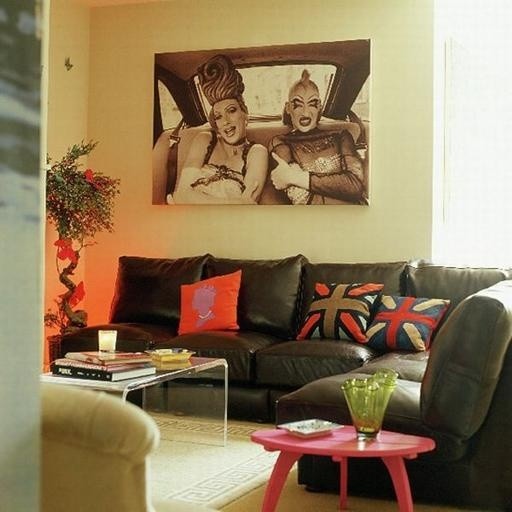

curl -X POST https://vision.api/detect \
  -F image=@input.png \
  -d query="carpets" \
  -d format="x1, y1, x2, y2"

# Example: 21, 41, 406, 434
139, 401, 298, 512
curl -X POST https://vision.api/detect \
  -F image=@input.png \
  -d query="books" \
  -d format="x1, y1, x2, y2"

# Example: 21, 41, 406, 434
51, 350, 158, 382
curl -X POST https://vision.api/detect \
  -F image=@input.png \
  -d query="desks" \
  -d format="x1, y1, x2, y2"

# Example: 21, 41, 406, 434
251, 419, 436, 511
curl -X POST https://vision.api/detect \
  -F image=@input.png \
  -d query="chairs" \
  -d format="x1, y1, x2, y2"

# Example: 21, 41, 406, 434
42, 385, 220, 511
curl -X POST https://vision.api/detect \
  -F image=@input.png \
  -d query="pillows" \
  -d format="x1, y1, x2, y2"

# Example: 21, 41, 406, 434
366, 292, 452, 353
295, 281, 385, 346
178, 267, 244, 336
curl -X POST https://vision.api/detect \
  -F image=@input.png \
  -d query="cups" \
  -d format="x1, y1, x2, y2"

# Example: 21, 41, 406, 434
98, 330, 117, 354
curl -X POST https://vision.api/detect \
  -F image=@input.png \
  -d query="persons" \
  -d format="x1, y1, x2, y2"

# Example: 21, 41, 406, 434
166, 54, 280, 205
269, 69, 366, 205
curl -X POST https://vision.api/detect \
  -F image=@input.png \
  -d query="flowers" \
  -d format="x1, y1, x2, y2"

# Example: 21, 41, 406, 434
44, 136, 124, 337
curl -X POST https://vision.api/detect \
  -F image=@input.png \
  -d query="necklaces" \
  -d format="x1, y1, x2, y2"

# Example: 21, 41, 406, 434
231, 147, 238, 156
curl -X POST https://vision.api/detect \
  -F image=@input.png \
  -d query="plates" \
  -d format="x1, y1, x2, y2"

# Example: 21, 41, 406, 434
150, 359, 193, 370
143, 347, 196, 361
277, 418, 346, 437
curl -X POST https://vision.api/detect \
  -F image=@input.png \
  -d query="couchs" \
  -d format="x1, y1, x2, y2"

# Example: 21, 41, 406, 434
70, 252, 512, 511
